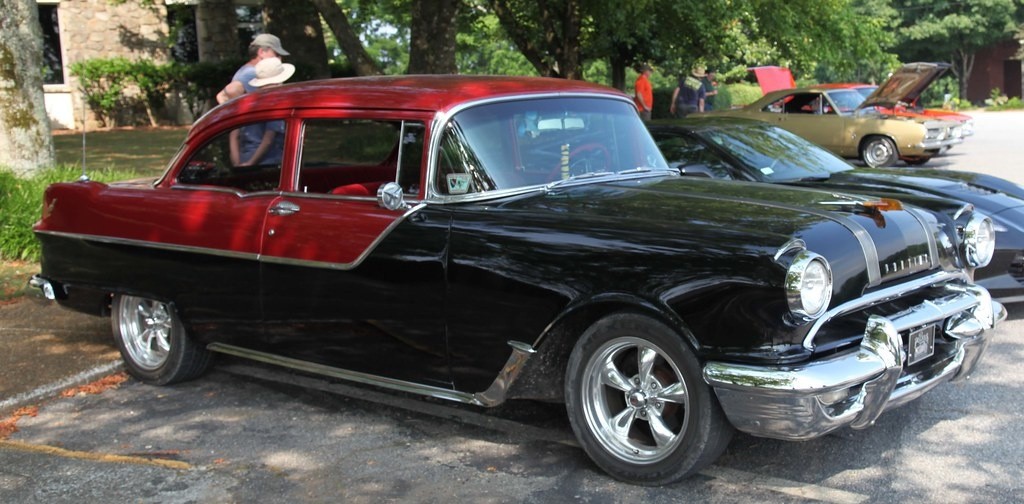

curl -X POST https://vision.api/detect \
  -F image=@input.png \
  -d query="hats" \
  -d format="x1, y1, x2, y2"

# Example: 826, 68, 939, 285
250, 34, 291, 55
640, 62, 652, 73
248, 57, 295, 86
692, 66, 707, 77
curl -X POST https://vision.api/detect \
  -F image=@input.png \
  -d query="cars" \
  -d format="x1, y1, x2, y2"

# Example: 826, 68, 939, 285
731, 58, 974, 169
29, 70, 1010, 491
518, 112, 1024, 309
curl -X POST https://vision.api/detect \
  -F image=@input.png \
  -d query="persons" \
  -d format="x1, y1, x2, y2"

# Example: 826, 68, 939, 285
216, 32, 296, 166
634, 65, 654, 120
670, 66, 707, 119
701, 68, 718, 111
